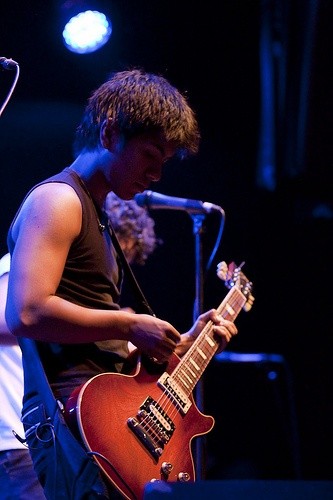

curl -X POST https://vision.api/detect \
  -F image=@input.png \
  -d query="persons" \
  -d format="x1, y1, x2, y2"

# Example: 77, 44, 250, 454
0, 189, 157, 500
4, 69, 239, 500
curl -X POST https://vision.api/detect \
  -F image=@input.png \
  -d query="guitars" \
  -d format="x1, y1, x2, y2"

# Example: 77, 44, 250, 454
63, 260, 257, 500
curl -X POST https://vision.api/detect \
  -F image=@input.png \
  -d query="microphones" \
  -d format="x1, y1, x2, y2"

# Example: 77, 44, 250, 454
0, 56, 19, 72
135, 190, 212, 214
215, 351, 283, 363
